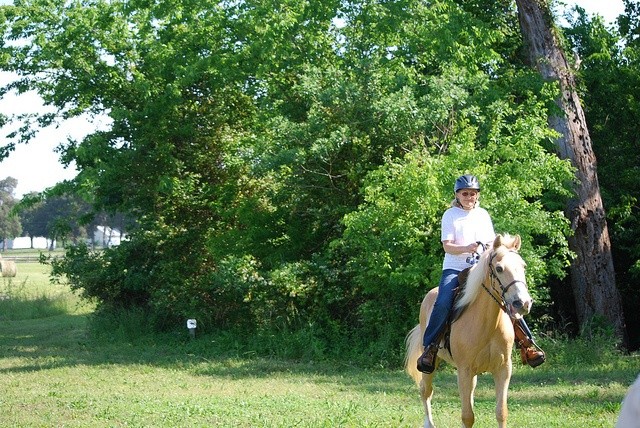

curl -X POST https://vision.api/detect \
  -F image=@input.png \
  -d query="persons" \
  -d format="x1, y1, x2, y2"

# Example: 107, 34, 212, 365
414, 173, 548, 376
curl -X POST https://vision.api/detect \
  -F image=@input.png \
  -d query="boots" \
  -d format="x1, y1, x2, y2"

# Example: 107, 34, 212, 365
518, 339, 546, 366
417, 342, 440, 375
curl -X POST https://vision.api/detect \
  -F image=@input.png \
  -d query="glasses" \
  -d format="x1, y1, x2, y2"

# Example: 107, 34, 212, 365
461, 192, 475, 196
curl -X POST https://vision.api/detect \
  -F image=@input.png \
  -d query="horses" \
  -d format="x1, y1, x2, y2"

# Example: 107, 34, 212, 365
403, 232, 535, 428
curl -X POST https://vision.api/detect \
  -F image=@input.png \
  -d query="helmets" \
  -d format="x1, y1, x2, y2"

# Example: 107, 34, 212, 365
454, 174, 480, 193
